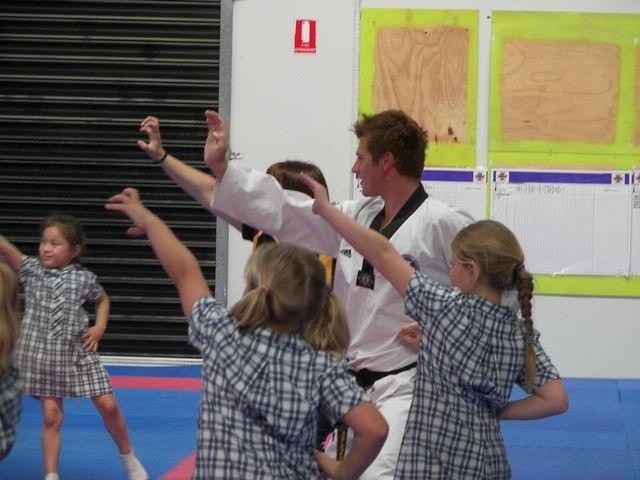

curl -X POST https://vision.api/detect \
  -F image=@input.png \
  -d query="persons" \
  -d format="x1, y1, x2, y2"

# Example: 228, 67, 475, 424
137, 115, 336, 291
299, 171, 570, 479
203, 109, 525, 477
1, 215, 151, 480
104, 187, 389, 479
0, 262, 24, 462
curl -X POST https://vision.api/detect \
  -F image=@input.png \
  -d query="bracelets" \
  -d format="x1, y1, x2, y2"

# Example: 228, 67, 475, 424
154, 151, 168, 165
